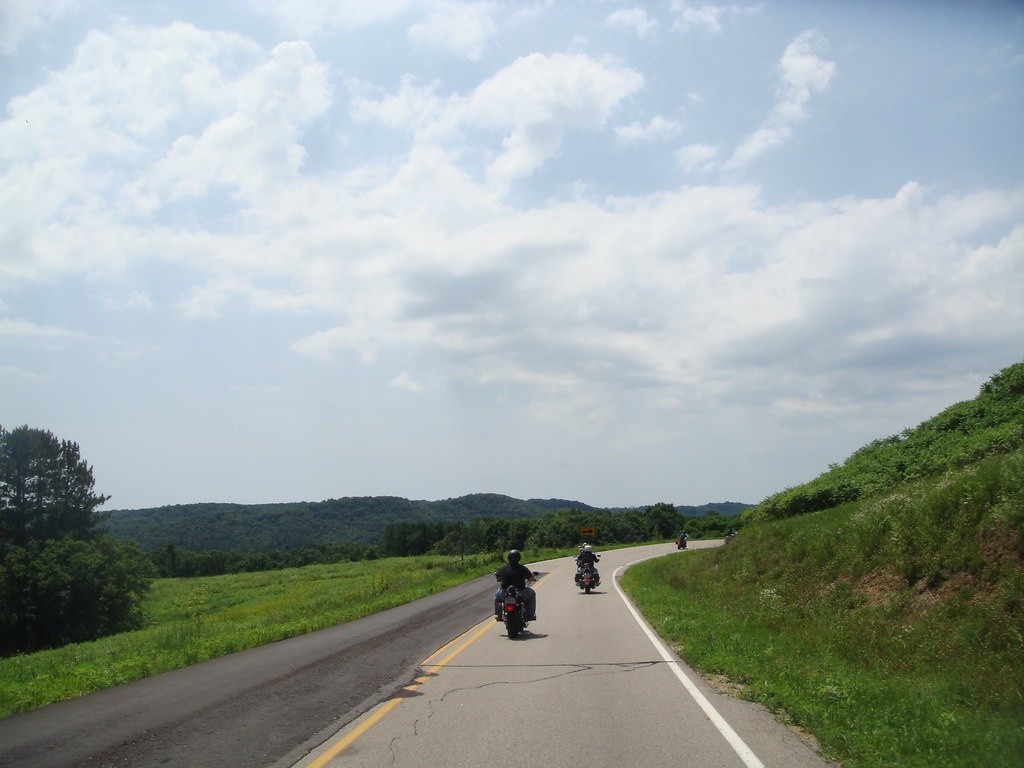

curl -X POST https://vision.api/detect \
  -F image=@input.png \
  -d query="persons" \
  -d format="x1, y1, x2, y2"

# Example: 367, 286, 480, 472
679, 531, 688, 547
495, 550, 536, 621
575, 543, 602, 586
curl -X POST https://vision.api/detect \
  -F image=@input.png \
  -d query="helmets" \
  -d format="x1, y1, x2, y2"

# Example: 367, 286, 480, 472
508, 549, 521, 561
584, 545, 592, 552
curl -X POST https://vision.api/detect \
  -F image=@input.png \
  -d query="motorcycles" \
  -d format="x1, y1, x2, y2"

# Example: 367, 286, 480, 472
675, 538, 687, 550
493, 571, 539, 640
573, 554, 601, 594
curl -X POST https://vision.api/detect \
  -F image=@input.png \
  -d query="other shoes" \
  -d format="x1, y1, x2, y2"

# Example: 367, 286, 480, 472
494, 615, 502, 620
528, 613, 537, 621
